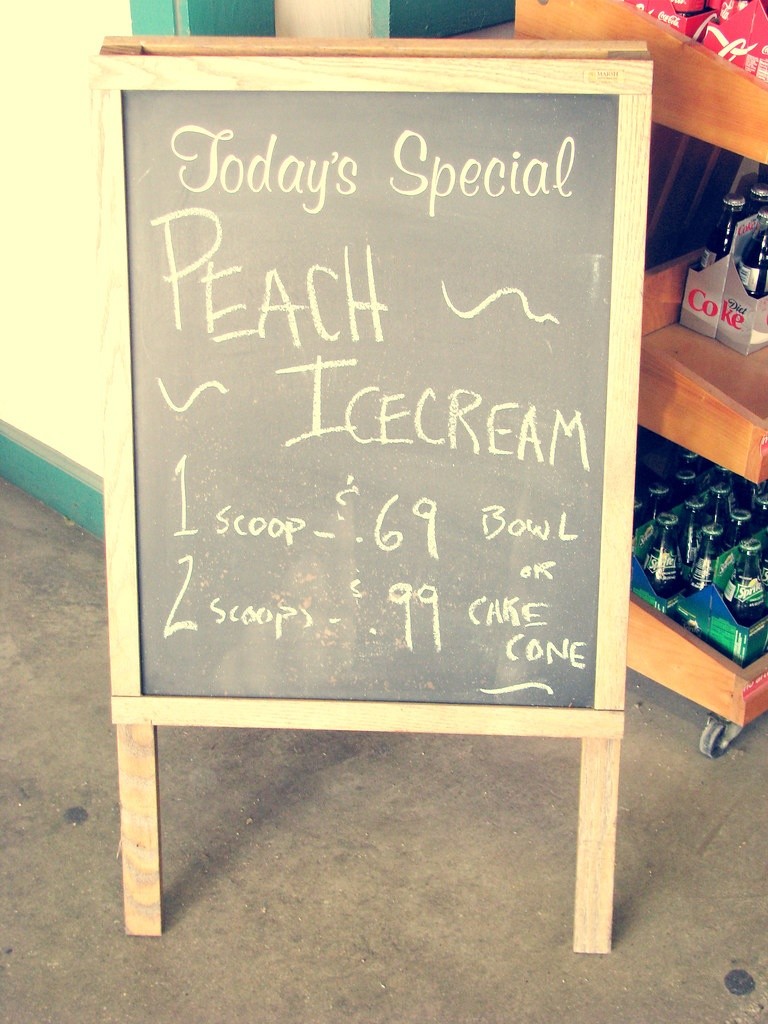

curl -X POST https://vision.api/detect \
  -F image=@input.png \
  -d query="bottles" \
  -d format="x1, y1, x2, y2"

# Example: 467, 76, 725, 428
700, 183, 767, 300
634, 423, 767, 667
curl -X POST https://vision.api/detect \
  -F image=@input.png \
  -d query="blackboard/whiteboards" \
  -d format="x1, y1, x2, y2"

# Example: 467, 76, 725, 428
91, 55, 653, 742
